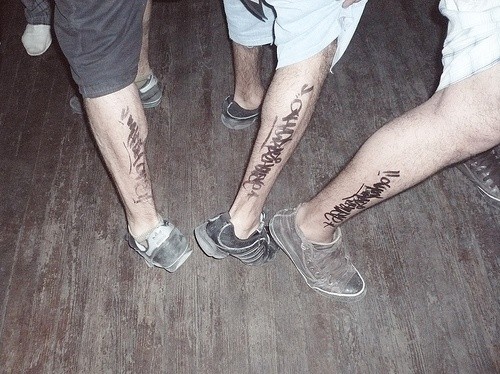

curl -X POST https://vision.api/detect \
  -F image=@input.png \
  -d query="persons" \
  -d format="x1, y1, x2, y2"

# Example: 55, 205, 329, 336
18, 0, 500, 299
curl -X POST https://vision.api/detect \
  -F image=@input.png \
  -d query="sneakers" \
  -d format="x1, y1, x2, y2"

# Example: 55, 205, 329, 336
269, 202, 366, 303
451, 144, 500, 210
195, 210, 280, 267
70, 73, 163, 114
21, 23, 52, 56
221, 93, 264, 130
125, 214, 194, 272
